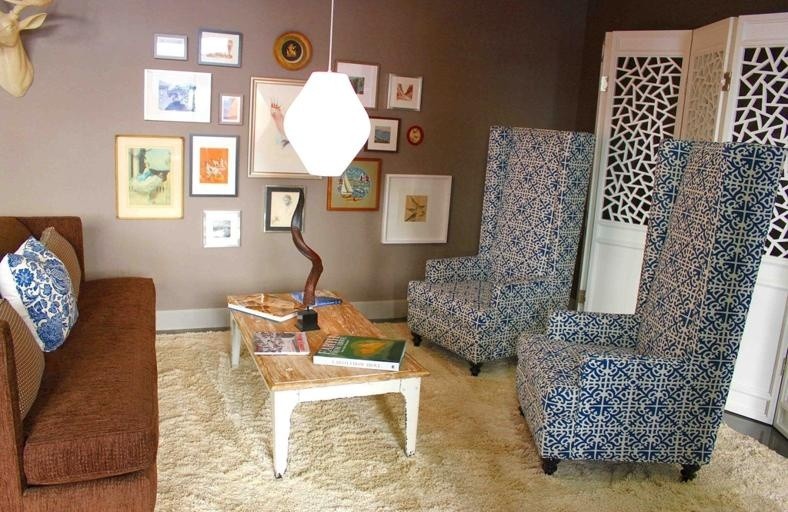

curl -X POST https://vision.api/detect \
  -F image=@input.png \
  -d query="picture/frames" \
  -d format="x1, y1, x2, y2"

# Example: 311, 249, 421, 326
201, 208, 242, 250
143, 69, 210, 124
152, 32, 190, 60
197, 27, 243, 69
221, 93, 243, 125
365, 115, 401, 152
262, 184, 307, 234
326, 157, 381, 212
188, 133, 241, 198
246, 76, 321, 181
386, 72, 423, 111
382, 174, 452, 246
114, 134, 185, 220
334, 59, 380, 111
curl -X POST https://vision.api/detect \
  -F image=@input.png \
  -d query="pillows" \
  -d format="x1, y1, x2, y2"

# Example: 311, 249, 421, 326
1, 296, 46, 420
41, 228, 80, 300
0, 237, 80, 352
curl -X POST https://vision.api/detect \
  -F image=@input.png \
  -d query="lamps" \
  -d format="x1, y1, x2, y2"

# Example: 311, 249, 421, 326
282, 0, 370, 177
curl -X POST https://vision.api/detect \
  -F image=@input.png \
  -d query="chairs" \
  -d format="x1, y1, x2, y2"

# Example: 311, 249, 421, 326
516, 140, 784, 481
408, 124, 597, 376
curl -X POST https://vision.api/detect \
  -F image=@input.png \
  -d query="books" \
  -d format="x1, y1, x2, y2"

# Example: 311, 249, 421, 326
252, 331, 310, 356
290, 289, 342, 307
313, 333, 407, 372
227, 292, 306, 323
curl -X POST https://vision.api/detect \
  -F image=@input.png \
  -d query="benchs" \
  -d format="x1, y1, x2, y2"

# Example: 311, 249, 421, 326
1, 217, 160, 512
